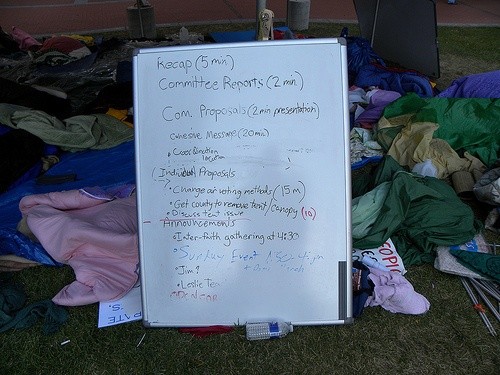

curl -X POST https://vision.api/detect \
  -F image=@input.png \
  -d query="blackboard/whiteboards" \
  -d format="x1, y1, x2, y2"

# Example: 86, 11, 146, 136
133, 38, 357, 327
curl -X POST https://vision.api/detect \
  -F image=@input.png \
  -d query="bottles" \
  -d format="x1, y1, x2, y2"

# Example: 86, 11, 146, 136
411, 159, 437, 179
246, 322, 294, 341
